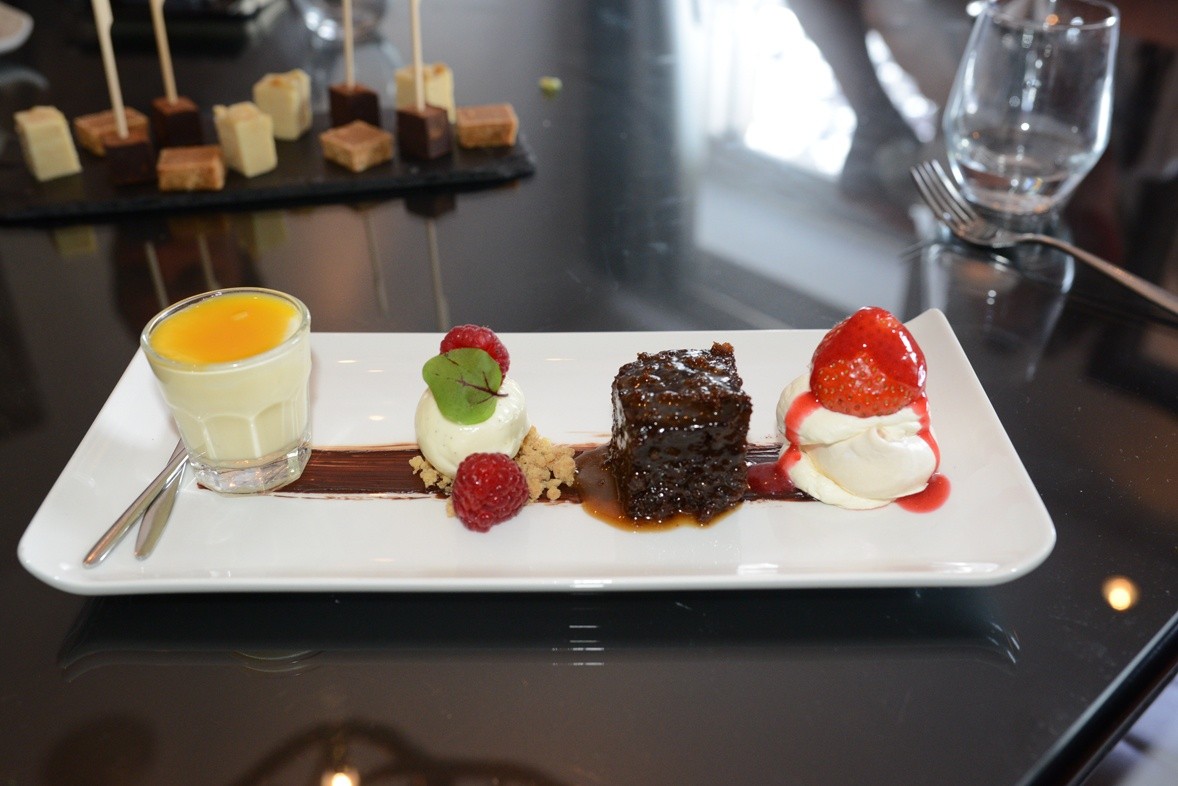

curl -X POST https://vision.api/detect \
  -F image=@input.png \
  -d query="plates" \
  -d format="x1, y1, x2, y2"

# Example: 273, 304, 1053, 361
18, 305, 1059, 594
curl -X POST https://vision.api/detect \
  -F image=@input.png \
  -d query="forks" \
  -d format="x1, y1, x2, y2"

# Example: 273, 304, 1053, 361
910, 157, 1176, 325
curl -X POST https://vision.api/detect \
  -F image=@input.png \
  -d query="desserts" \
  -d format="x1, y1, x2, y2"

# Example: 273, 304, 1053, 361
76, 62, 457, 190
775, 306, 940, 513
141, 287, 309, 471
453, 103, 520, 149
416, 303, 577, 533
15, 107, 84, 180
610, 343, 752, 524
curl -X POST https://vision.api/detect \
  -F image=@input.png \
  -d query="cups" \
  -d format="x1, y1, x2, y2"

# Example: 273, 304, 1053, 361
140, 285, 318, 499
939, 0, 1122, 217
